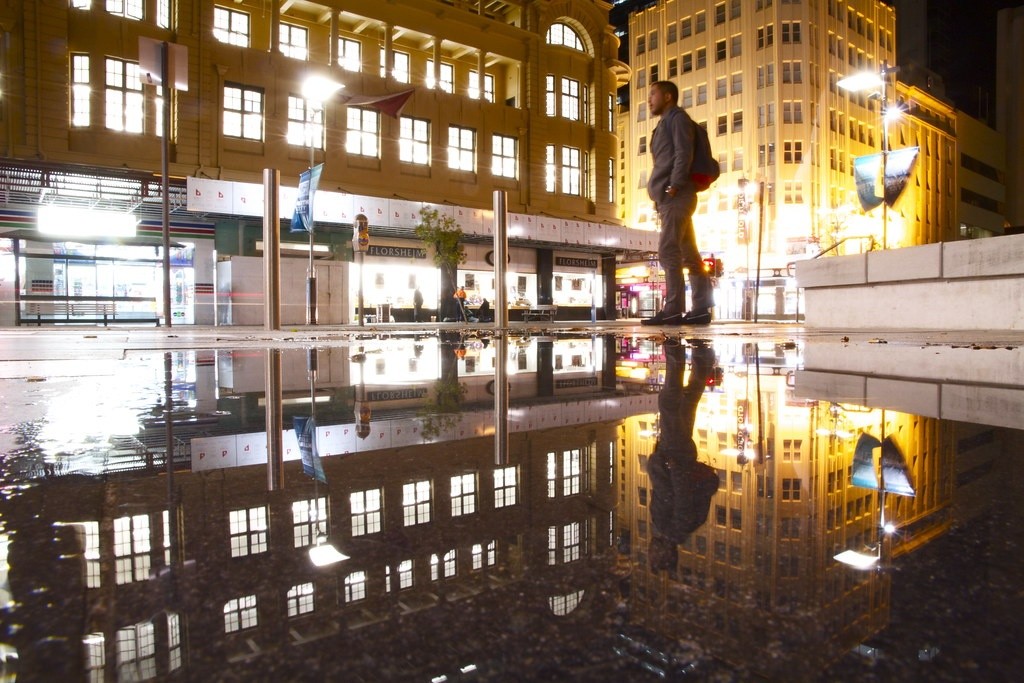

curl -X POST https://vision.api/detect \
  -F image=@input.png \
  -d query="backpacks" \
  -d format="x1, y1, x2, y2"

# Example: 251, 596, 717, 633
667, 106, 720, 192
667, 461, 719, 545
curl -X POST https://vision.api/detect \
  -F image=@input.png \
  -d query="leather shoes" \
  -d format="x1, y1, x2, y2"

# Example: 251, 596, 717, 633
685, 339, 714, 349
647, 334, 681, 347
641, 309, 682, 325
681, 311, 712, 324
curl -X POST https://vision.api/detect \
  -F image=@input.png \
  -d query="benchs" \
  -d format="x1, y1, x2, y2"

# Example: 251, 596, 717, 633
521, 304, 557, 324
25, 302, 117, 326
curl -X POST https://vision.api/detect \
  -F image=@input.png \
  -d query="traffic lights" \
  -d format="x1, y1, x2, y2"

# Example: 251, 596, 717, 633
704, 367, 724, 388
703, 256, 723, 278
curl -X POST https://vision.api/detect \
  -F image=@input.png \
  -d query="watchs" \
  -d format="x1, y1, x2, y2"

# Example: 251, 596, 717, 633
665, 186, 676, 196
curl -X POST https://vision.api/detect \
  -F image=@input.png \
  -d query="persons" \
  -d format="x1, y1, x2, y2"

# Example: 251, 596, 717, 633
413, 286, 423, 323
478, 298, 492, 322
453, 286, 466, 322
464, 306, 479, 323
641, 81, 720, 326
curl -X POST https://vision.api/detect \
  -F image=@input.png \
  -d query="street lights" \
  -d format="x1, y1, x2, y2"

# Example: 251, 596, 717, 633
836, 61, 906, 252
307, 349, 349, 568
832, 407, 898, 569
302, 68, 346, 325
